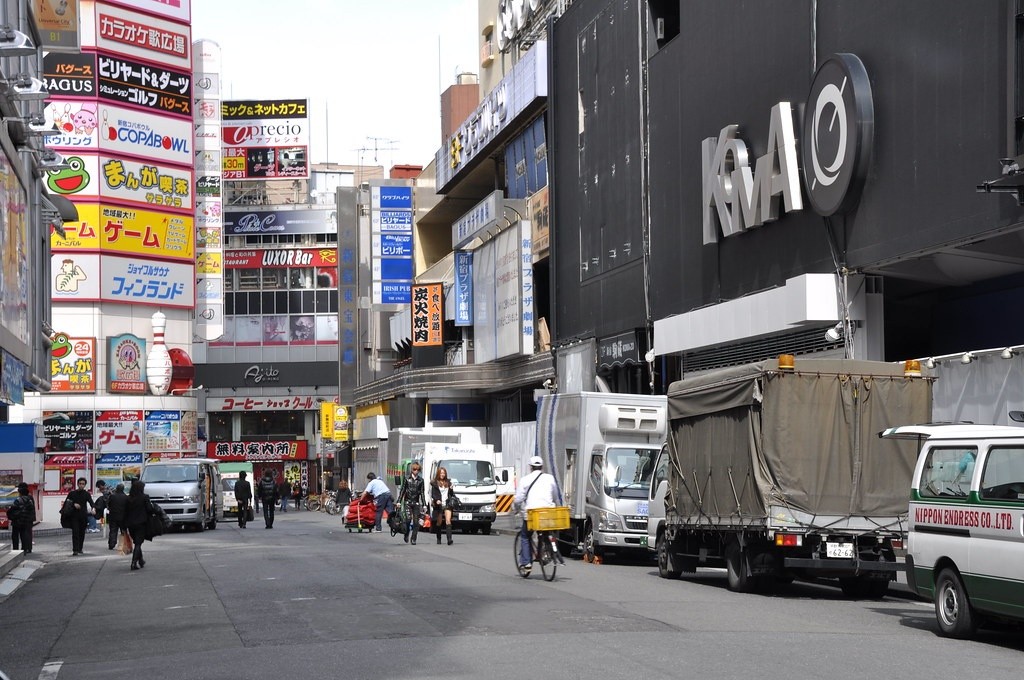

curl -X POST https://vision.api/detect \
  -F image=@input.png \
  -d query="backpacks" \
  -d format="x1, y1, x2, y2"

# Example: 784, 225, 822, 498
19, 498, 34, 523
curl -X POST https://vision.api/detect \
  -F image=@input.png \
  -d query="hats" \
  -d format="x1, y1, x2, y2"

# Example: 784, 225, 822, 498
528, 456, 544, 466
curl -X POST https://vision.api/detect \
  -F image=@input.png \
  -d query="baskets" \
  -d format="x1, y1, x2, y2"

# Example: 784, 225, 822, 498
526, 504, 570, 531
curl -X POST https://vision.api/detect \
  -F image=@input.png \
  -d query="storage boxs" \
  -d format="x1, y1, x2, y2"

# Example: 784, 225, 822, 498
526, 507, 571, 531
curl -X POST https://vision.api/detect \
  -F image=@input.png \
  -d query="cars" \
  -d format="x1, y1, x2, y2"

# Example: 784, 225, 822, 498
0, 500, 14, 529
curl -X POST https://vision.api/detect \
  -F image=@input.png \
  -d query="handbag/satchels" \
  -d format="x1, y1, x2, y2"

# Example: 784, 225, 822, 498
390, 508, 406, 537
446, 490, 462, 510
122, 531, 132, 554
150, 501, 172, 536
245, 500, 254, 521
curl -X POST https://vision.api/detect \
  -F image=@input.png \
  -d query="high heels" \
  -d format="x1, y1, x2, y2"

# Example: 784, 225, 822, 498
131, 562, 139, 570
139, 560, 145, 568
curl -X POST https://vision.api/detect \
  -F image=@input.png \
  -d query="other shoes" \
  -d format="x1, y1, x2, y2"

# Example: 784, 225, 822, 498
265, 525, 273, 529
524, 563, 532, 571
73, 549, 83, 555
411, 535, 417, 544
376, 526, 382, 532
404, 531, 409, 543
109, 545, 114, 550
238, 522, 246, 528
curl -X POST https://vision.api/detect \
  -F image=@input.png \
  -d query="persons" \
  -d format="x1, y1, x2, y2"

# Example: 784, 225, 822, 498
431, 467, 455, 545
511, 456, 557, 571
125, 478, 171, 570
396, 462, 427, 545
12, 482, 36, 556
280, 478, 291, 512
66, 478, 96, 555
336, 480, 351, 523
293, 480, 303, 510
361, 472, 394, 532
254, 470, 280, 529
234, 471, 252, 528
88, 479, 127, 550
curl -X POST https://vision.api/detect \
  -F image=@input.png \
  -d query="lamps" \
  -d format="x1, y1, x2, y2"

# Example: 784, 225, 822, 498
36, 147, 56, 161
41, 193, 66, 238
7, 73, 31, 88
24, 112, 45, 126
0, 24, 16, 42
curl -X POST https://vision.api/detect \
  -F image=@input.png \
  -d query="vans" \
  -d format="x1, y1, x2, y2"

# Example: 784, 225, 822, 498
876, 411, 1024, 640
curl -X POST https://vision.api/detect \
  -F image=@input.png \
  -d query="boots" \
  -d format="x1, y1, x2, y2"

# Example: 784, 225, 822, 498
445, 523, 453, 545
435, 524, 443, 544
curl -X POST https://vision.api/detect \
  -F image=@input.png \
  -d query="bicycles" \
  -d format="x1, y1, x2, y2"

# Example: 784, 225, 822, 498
303, 490, 342, 516
512, 507, 561, 583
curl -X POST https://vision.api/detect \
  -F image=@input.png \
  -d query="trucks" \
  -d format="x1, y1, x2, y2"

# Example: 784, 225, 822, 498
640, 354, 939, 595
218, 462, 258, 516
401, 441, 509, 536
535, 390, 669, 566
138, 458, 223, 532
